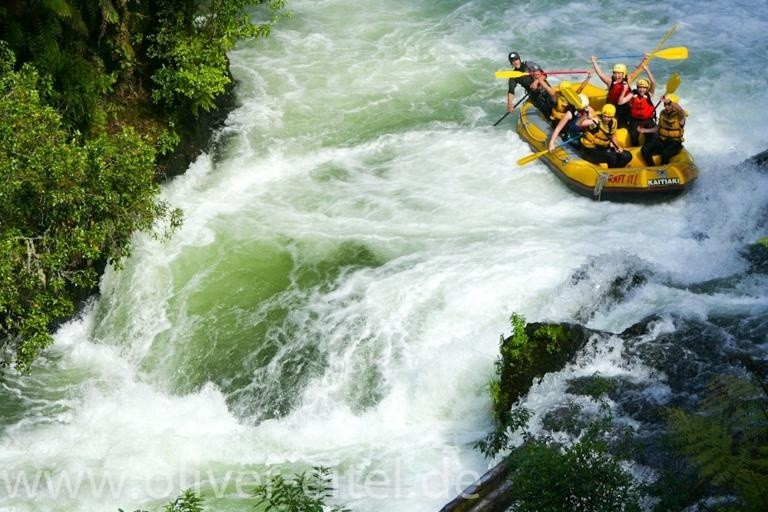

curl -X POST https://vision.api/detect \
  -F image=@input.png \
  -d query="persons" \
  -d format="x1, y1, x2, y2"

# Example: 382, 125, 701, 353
589, 51, 651, 131
537, 71, 593, 139
636, 90, 685, 166
579, 102, 632, 168
616, 63, 657, 148
506, 51, 554, 120
548, 94, 596, 155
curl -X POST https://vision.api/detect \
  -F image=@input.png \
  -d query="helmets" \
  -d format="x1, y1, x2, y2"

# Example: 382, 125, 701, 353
613, 64, 628, 78
663, 93, 679, 107
637, 78, 651, 92
601, 103, 617, 117
509, 52, 520, 64
560, 80, 571, 89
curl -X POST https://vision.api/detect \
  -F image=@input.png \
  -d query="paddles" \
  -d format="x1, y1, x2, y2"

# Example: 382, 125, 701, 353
590, 47, 687, 60
640, 71, 682, 128
516, 134, 585, 165
560, 88, 619, 150
632, 24, 678, 81
495, 71, 589, 78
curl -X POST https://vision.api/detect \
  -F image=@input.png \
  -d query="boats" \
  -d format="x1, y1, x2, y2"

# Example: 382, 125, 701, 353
516, 82, 701, 205
